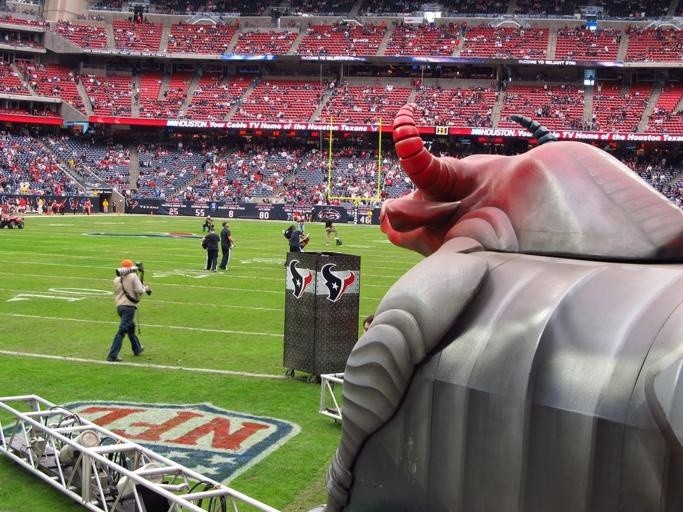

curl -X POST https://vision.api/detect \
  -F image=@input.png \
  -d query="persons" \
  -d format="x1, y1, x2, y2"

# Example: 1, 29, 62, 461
203, 230, 220, 272
288, 226, 303, 252
106, 258, 152, 362
0, 0, 683, 232
362, 315, 374, 334
219, 222, 236, 270
324, 213, 338, 246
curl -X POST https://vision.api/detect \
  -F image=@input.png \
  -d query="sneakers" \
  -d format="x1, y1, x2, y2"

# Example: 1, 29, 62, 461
106, 356, 123, 362
134, 345, 144, 356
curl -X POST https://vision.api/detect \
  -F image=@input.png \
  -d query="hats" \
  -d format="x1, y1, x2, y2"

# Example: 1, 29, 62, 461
121, 259, 132, 267
